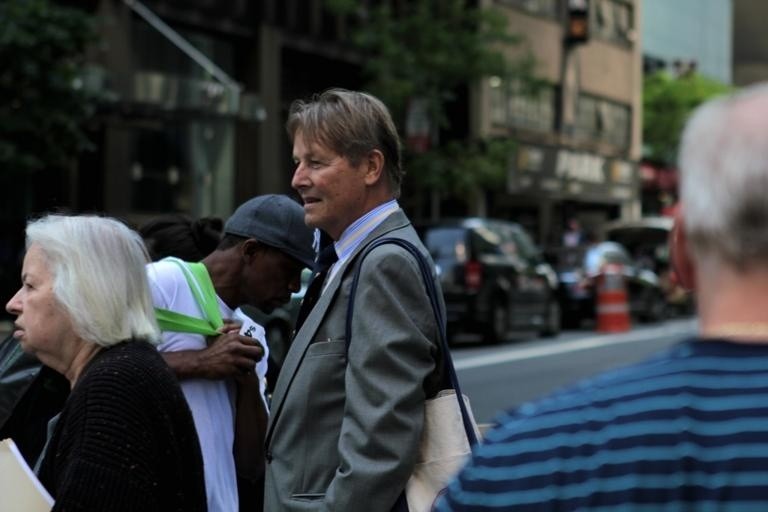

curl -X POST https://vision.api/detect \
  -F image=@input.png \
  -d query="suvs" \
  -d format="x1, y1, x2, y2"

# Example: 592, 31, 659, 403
407, 216, 569, 346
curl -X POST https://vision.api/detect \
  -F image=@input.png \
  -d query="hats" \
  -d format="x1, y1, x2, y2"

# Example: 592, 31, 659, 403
224, 193, 316, 271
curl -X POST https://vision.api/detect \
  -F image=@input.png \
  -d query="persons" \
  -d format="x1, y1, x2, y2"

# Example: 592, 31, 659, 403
5, 210, 207, 512
142, 191, 319, 511
261, 83, 456, 512
426, 78, 768, 512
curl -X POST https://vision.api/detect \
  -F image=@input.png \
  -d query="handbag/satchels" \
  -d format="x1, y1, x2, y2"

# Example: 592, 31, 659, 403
406, 388, 482, 512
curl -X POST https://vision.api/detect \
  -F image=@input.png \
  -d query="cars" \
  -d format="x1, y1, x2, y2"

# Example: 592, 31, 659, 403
240, 267, 313, 376
562, 217, 693, 322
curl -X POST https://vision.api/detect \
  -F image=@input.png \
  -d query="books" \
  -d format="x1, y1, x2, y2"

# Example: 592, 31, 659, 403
0, 433, 60, 512
